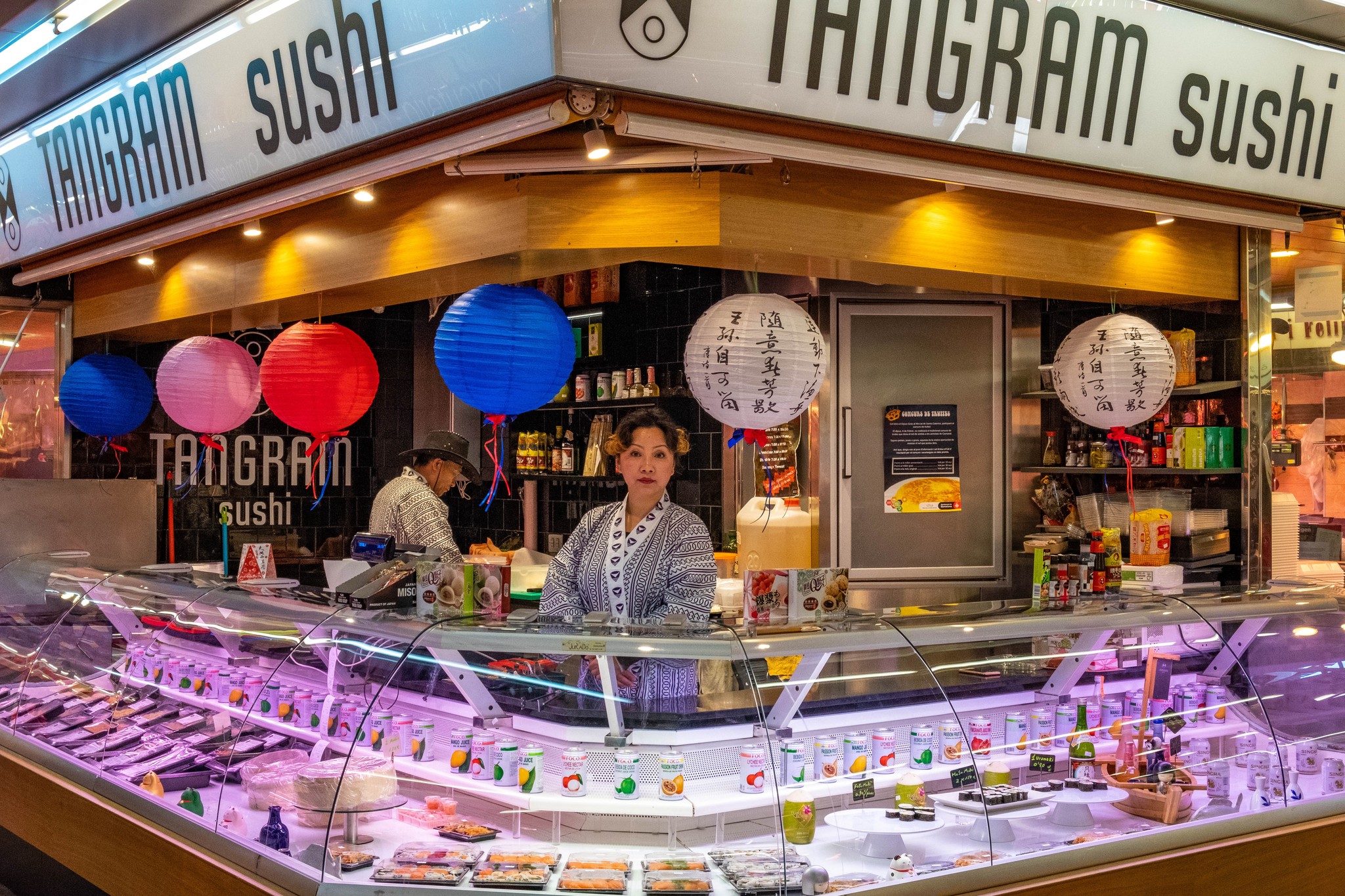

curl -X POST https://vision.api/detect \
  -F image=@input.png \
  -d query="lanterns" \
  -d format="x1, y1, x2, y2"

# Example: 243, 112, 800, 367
259, 292, 379, 511
683, 263, 827, 533
1052, 290, 1177, 520
155, 315, 262, 511
433, 260, 577, 511
58, 334, 154, 497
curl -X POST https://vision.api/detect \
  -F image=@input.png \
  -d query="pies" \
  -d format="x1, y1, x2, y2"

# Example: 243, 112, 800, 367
896, 477, 961, 511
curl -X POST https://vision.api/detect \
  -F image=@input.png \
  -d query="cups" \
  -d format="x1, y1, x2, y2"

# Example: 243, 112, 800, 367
1127, 779, 1146, 790
399, 795, 458, 821
783, 789, 816, 845
1038, 364, 1055, 392
1173, 781, 1190, 792
893, 772, 926, 808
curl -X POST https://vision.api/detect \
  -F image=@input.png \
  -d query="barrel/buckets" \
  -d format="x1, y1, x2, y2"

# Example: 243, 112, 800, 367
735, 496, 812, 579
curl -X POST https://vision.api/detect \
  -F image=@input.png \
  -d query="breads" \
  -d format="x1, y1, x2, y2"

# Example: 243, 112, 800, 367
295, 757, 397, 810
239, 750, 349, 828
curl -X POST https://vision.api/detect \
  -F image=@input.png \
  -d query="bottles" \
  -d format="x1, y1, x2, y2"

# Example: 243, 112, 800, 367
1048, 531, 1105, 609
1146, 718, 1177, 792
660, 369, 693, 398
1089, 675, 1105, 740
259, 805, 289, 853
621, 366, 660, 399
1068, 697, 1096, 780
1250, 770, 1304, 811
981, 760, 1012, 788
1116, 716, 1140, 782
1042, 412, 1174, 468
552, 409, 579, 476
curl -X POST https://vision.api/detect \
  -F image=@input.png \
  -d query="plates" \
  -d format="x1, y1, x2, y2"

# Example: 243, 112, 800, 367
1271, 491, 1300, 580
341, 825, 810, 896
0, 682, 316, 777
927, 784, 1056, 814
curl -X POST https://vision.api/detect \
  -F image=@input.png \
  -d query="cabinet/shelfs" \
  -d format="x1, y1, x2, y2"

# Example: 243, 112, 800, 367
509, 394, 686, 493
1012, 379, 1241, 571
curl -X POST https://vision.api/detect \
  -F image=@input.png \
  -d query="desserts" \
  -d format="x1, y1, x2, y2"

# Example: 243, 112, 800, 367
820, 575, 848, 610
439, 566, 500, 604
751, 570, 789, 610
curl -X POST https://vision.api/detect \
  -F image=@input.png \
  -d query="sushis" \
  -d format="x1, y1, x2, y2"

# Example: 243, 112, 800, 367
1031, 778, 1108, 792
473, 852, 554, 882
710, 847, 809, 889
958, 784, 1028, 806
559, 861, 628, 889
374, 848, 478, 881
885, 804, 935, 821
647, 860, 709, 890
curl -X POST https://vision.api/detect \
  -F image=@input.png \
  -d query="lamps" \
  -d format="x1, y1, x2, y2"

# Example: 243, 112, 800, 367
242, 218, 264, 240
1152, 208, 1178, 227
134, 249, 157, 268
939, 180, 970, 195
349, 184, 377, 204
578, 117, 611, 160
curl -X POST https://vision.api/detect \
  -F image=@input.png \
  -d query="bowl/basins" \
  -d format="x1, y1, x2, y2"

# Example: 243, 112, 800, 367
1023, 540, 1068, 554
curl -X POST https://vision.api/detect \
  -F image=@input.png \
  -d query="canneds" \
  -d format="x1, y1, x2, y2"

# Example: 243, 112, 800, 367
1176, 730, 1345, 800
575, 375, 591, 402
1089, 442, 1109, 468
597, 373, 611, 400
449, 728, 545, 794
909, 708, 1052, 770
561, 747, 684, 801
1055, 683, 1226, 748
739, 728, 895, 793
611, 371, 626, 399
125, 641, 435, 762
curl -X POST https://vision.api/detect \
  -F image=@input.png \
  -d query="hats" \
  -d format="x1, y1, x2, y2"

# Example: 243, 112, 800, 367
398, 430, 482, 486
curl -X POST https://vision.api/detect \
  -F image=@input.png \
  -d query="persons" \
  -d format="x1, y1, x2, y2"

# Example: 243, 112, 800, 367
369, 429, 483, 570
535, 407, 717, 713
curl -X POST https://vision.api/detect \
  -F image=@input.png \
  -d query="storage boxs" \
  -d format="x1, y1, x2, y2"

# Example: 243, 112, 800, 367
334, 558, 515, 623
1032, 546, 1052, 602
1171, 425, 1235, 471
741, 566, 851, 623
